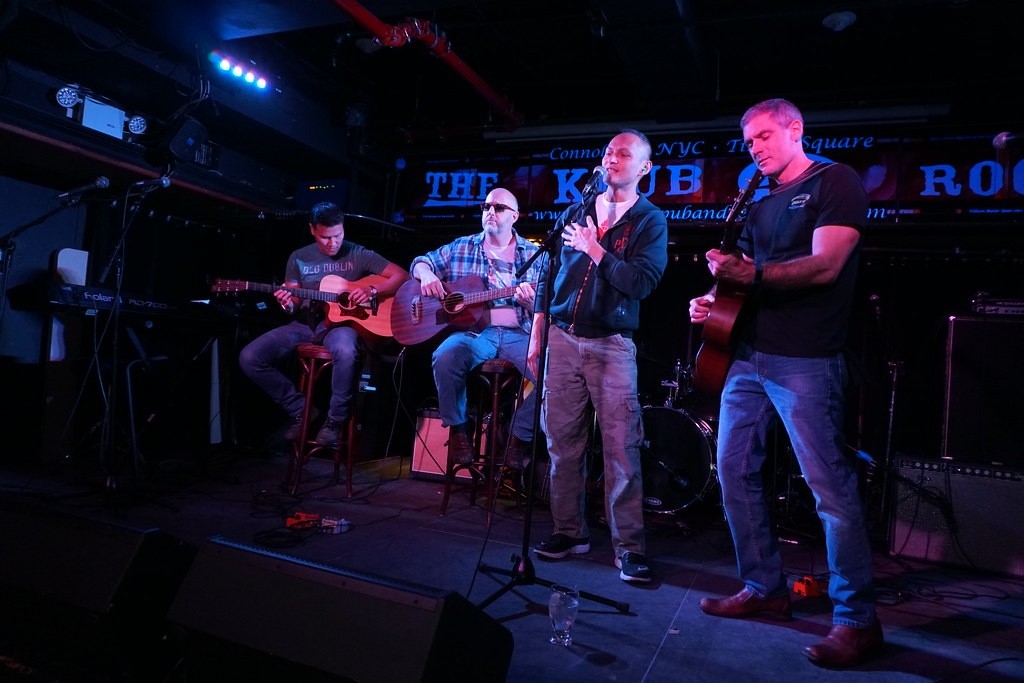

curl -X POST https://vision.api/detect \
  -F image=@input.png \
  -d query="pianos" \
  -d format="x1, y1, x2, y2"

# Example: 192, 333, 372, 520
47, 281, 251, 474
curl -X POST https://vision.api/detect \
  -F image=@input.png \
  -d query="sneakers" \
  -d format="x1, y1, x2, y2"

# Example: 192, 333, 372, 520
614, 550, 651, 581
533, 531, 590, 558
285, 406, 319, 440
316, 417, 345, 445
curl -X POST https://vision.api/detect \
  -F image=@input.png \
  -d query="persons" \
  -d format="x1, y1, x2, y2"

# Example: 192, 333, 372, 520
687, 100, 882, 665
410, 187, 548, 466
240, 202, 410, 446
527, 130, 668, 582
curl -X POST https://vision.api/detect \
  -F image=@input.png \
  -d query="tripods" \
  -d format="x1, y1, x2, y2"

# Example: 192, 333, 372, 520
478, 186, 631, 616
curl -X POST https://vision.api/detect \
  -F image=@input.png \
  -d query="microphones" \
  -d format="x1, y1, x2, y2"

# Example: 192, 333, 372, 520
134, 177, 171, 188
51, 176, 109, 200
677, 479, 690, 488
945, 503, 956, 530
582, 166, 606, 196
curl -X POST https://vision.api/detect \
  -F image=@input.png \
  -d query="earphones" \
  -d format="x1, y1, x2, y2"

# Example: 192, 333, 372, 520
642, 166, 646, 171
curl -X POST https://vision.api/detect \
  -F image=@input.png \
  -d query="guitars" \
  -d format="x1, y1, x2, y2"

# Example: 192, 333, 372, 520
690, 167, 766, 396
209, 272, 399, 348
388, 274, 537, 351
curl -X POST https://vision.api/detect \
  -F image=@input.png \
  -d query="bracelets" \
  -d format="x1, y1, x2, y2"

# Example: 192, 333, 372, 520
754, 264, 762, 285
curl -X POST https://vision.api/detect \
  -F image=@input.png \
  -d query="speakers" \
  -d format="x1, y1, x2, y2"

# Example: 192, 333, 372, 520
0, 495, 515, 683
166, 116, 206, 162
940, 316, 1024, 472
890, 454, 1024, 579
410, 409, 490, 488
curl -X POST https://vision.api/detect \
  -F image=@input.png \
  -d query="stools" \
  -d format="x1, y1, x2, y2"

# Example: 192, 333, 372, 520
289, 343, 356, 501
441, 359, 525, 522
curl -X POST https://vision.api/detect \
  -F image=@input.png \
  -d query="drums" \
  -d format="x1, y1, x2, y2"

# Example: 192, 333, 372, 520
678, 363, 721, 422
639, 403, 719, 516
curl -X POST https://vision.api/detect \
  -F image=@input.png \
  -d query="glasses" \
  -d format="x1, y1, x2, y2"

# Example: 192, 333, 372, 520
480, 203, 515, 213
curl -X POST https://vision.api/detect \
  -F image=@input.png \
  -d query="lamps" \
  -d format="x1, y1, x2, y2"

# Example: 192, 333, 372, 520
56, 87, 149, 140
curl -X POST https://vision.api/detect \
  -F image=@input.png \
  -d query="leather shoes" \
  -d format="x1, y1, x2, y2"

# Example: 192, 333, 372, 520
505, 434, 528, 470
801, 617, 884, 666
699, 585, 792, 621
448, 426, 472, 464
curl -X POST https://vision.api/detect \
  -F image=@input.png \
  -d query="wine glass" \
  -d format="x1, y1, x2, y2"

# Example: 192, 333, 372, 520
547, 582, 581, 648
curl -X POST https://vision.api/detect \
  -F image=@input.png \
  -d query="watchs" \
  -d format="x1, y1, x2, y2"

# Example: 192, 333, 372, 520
370, 286, 378, 296
